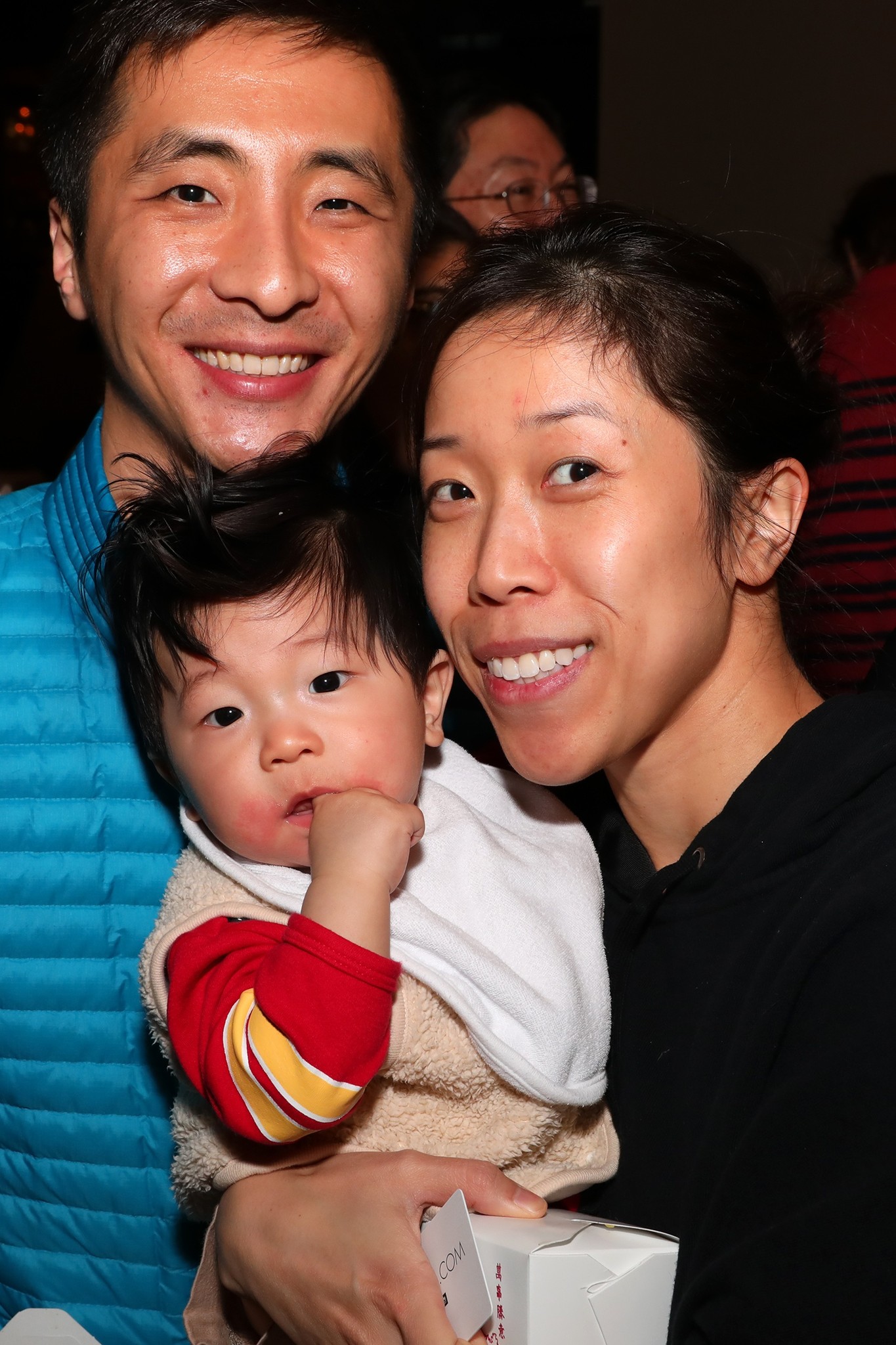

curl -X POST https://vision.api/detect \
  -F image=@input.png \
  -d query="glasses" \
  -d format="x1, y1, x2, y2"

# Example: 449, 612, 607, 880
444, 176, 596, 221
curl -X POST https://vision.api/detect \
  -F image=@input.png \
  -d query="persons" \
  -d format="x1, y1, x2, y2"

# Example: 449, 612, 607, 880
784, 168, 896, 688
185, 201, 896, 1345
0, 0, 434, 1345
405, 79, 597, 305
95, 437, 620, 1205
